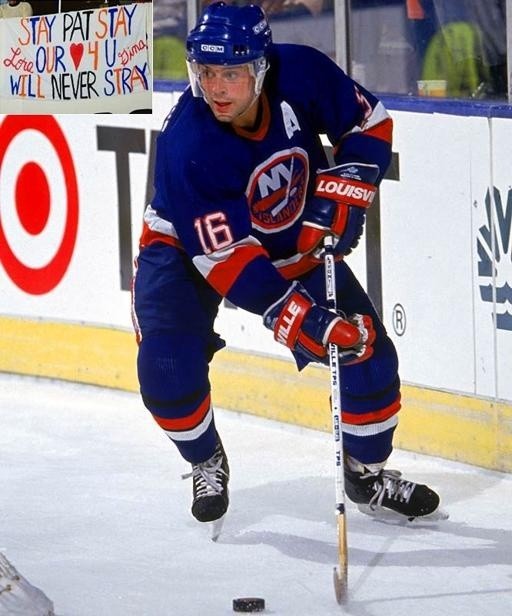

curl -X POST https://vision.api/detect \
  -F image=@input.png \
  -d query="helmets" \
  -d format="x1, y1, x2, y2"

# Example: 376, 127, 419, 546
186, 3, 271, 98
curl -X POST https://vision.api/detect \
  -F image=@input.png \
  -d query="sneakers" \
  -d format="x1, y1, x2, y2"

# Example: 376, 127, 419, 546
344, 455, 440, 516
192, 433, 230, 522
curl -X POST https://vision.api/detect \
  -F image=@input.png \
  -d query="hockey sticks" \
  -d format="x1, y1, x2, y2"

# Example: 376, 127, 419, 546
324, 232, 349, 604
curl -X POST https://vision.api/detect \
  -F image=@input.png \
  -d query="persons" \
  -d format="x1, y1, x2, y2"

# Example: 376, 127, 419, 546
0, 0, 34, 20
131, 1, 448, 525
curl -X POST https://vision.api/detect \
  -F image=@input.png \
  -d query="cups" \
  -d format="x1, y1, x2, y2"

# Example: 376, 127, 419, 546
415, 78, 450, 97
352, 62, 367, 87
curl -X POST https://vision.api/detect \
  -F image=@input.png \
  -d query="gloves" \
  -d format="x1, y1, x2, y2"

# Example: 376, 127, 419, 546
263, 281, 376, 366
296, 161, 381, 262
